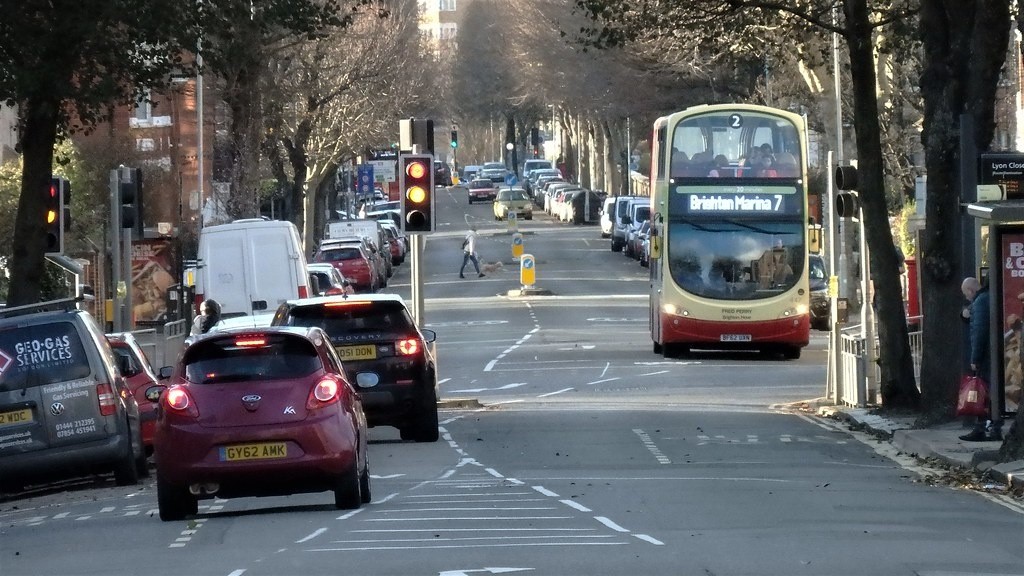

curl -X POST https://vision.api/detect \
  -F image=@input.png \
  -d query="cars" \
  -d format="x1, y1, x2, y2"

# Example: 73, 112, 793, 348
432, 161, 453, 188
523, 159, 651, 267
808, 254, 834, 334
267, 291, 440, 443
470, 161, 508, 183
493, 188, 532, 220
200, 312, 279, 331
146, 324, 381, 523
464, 178, 502, 204
304, 186, 419, 296
103, 332, 174, 476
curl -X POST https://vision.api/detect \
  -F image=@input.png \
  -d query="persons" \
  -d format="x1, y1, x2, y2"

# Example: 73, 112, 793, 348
958, 275, 992, 442
458, 225, 485, 279
748, 142, 777, 172
183, 298, 226, 380
769, 233, 794, 277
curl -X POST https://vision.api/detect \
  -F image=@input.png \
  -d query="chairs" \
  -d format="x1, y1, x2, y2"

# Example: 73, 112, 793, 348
778, 151, 797, 165
672, 152, 730, 173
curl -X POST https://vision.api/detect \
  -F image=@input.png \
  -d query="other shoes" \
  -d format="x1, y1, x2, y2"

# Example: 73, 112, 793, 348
460, 275, 465, 278
479, 273, 485, 277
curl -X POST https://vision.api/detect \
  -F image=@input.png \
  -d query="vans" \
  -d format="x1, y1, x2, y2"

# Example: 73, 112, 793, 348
194, 216, 315, 329
0, 295, 148, 495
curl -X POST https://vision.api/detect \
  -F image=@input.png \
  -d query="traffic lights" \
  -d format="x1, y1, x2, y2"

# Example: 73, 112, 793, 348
836, 163, 861, 218
533, 145, 539, 156
452, 131, 457, 149
116, 172, 135, 233
399, 154, 436, 235
47, 177, 62, 252
63, 178, 71, 233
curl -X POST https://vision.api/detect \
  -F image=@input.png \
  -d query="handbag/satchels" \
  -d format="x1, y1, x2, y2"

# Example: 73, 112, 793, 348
461, 235, 472, 249
957, 369, 990, 416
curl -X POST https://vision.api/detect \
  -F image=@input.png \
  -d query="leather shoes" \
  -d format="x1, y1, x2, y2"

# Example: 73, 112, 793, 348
958, 426, 1003, 441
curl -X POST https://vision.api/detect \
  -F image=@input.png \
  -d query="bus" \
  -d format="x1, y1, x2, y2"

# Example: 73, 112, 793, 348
646, 103, 822, 361
